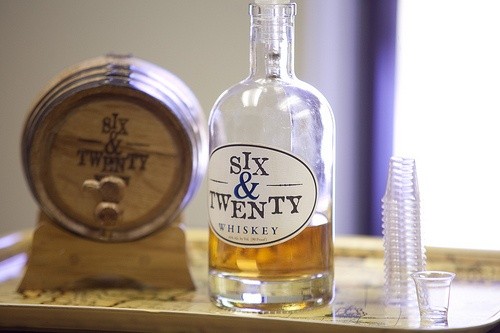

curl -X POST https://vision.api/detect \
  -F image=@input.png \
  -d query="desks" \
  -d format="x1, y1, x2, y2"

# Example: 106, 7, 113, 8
0, 228, 500, 333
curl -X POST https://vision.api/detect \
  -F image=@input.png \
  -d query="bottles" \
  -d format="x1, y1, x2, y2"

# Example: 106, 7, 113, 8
202, 1, 336, 312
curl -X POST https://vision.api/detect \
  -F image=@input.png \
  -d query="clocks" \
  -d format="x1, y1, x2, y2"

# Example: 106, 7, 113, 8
18, 54, 208, 296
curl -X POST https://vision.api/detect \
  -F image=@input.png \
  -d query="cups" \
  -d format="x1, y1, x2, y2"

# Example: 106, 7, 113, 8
381, 156, 425, 304
411, 271, 454, 322
